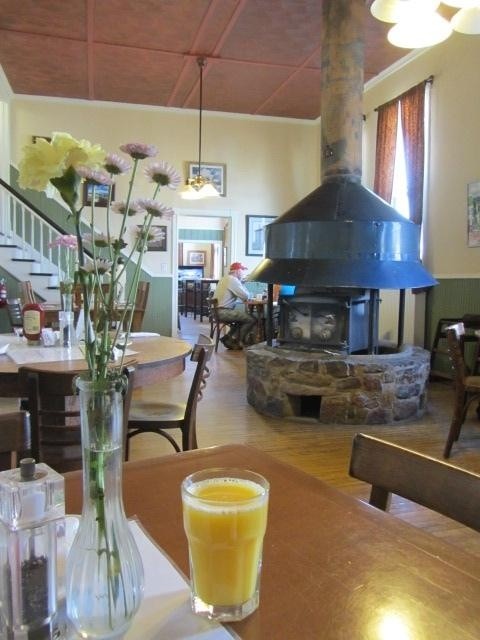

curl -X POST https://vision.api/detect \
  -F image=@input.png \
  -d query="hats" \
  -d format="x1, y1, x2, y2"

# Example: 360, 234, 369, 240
230, 263, 248, 270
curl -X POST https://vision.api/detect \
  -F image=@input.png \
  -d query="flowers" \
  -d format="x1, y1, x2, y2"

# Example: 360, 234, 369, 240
12, 130, 185, 630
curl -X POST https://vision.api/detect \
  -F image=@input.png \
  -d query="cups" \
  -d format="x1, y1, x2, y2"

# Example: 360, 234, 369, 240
177, 466, 271, 622
40, 328, 56, 347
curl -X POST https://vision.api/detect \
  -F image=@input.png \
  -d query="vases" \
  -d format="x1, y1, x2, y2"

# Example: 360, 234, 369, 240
60, 374, 147, 640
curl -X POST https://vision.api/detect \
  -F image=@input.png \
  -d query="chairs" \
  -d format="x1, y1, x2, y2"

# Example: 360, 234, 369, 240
127, 328, 216, 464
343, 433, 480, 539
435, 315, 480, 459
205, 298, 241, 351
8, 280, 150, 332
16, 361, 137, 480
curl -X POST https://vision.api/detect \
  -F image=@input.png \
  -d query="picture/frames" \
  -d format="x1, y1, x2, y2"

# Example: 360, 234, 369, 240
183, 162, 230, 198
134, 222, 167, 251
242, 213, 280, 257
83, 176, 114, 204
190, 250, 207, 265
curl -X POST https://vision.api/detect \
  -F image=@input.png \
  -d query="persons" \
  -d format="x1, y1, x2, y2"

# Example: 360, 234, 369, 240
273, 284, 295, 302
211, 263, 257, 350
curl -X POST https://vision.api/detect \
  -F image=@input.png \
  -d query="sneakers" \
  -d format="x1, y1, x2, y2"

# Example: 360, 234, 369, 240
220, 335, 244, 350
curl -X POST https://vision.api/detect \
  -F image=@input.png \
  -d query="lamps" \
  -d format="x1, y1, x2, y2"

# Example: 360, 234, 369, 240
371, 0, 480, 49
181, 56, 220, 202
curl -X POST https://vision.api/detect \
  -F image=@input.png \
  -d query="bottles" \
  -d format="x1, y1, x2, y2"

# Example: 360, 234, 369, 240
6, 297, 24, 338
0, 455, 67, 640
59, 311, 75, 348
